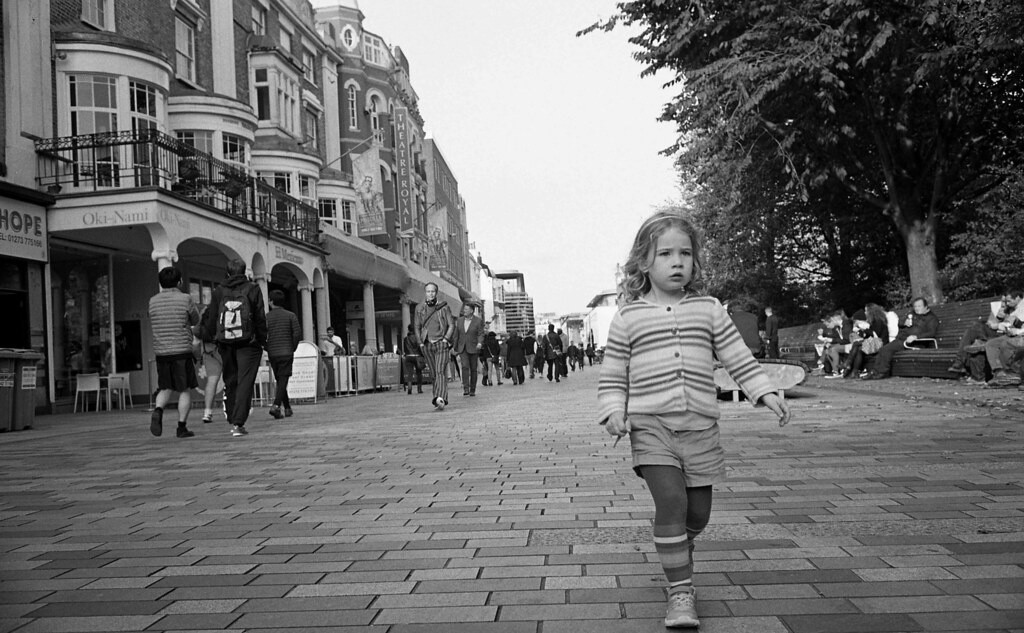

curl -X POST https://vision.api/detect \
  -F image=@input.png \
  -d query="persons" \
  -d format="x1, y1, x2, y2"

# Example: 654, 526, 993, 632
949, 292, 1024, 386
148, 257, 341, 439
404, 281, 594, 409
598, 214, 793, 628
724, 295, 937, 383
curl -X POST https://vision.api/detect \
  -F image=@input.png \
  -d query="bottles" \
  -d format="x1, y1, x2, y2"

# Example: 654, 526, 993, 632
908, 314, 913, 325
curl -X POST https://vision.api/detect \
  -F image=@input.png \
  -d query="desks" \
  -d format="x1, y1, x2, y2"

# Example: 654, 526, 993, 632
99, 376, 127, 411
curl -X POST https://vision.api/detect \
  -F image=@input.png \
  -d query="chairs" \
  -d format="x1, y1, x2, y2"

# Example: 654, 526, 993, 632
107, 372, 134, 411
74, 373, 109, 413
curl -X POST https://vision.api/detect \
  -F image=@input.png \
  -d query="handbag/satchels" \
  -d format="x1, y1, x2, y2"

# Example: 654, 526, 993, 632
416, 357, 426, 369
862, 331, 883, 354
553, 345, 563, 357
492, 356, 499, 364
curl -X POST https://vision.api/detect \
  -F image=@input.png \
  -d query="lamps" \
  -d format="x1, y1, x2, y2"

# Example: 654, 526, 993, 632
51, 41, 67, 62
452, 231, 457, 237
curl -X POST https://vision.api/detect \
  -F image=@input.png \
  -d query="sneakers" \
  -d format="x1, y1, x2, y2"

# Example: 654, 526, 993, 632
665, 587, 700, 628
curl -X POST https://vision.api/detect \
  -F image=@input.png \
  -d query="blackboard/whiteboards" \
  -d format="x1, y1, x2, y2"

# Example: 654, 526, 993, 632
375, 352, 398, 384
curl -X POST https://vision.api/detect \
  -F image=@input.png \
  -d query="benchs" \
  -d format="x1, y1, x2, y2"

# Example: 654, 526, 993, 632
775, 294, 1002, 380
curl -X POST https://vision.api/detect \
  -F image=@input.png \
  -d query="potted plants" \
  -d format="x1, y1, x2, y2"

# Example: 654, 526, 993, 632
219, 169, 247, 199
180, 159, 201, 180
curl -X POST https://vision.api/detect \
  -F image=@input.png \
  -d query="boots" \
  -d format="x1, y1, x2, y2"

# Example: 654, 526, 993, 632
417, 384, 423, 393
407, 381, 412, 395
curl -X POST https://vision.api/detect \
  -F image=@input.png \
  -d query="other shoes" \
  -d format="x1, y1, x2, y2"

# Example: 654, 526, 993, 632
837, 360, 850, 369
176, 421, 195, 438
149, 407, 163, 436
862, 371, 889, 380
230, 425, 249, 437
824, 369, 839, 378
463, 389, 475, 396
498, 380, 504, 385
988, 371, 1021, 385
269, 406, 292, 418
201, 409, 213, 422
431, 396, 446, 410
948, 360, 966, 372
961, 376, 985, 385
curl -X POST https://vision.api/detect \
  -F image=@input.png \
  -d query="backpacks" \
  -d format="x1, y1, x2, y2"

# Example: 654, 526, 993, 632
215, 281, 254, 346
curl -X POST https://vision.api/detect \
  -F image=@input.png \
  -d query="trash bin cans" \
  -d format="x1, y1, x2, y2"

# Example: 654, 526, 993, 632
0, 348, 45, 431
